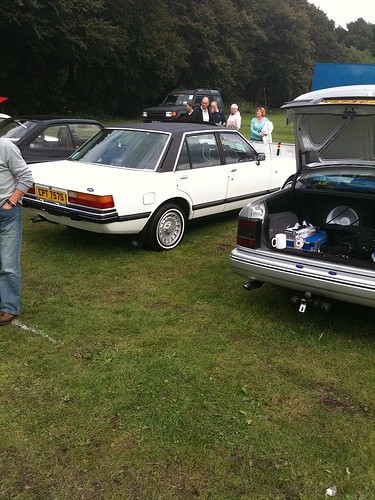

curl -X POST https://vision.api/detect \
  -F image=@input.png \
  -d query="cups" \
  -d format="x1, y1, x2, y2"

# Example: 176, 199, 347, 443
271, 234, 286, 249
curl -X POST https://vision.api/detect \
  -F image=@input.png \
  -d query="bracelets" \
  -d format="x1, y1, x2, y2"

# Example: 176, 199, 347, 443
6, 199, 15, 208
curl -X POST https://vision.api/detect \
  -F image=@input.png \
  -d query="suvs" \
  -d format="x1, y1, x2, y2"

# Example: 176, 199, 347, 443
143, 88, 224, 125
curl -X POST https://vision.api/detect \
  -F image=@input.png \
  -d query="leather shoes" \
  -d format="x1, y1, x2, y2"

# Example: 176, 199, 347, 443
0, 311, 18, 325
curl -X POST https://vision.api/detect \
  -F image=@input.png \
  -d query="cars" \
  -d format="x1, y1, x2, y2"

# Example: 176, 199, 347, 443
230, 84, 375, 314
16, 124, 296, 252
0, 113, 59, 141
0, 120, 103, 164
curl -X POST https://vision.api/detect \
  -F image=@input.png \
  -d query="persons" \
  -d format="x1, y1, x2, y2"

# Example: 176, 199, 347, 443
178, 97, 227, 129
250, 107, 274, 143
0, 137, 34, 325
227, 103, 242, 131
